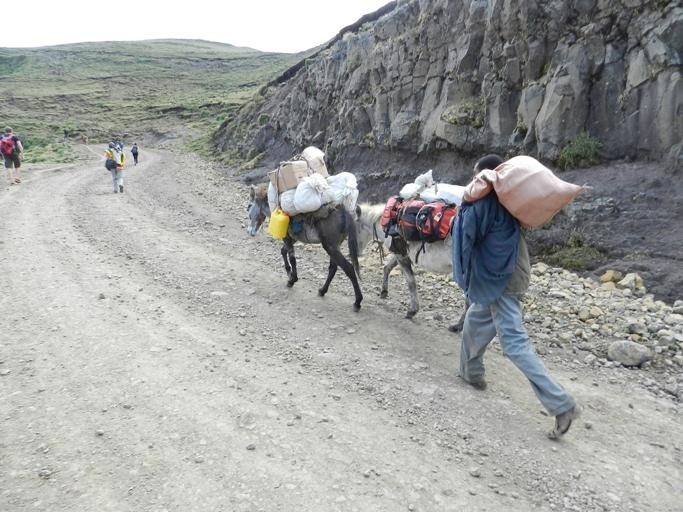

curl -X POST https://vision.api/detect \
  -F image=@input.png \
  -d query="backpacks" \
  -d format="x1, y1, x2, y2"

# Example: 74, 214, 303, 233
380, 196, 460, 243
0, 133, 16, 156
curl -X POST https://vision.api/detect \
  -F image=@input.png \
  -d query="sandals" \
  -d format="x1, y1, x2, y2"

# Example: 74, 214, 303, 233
470, 375, 489, 392
544, 404, 584, 441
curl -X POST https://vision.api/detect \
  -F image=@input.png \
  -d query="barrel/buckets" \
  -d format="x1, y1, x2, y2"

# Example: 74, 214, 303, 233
267, 208, 289, 242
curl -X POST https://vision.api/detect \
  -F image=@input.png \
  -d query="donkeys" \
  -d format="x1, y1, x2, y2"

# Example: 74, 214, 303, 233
245, 182, 363, 312
354, 199, 471, 334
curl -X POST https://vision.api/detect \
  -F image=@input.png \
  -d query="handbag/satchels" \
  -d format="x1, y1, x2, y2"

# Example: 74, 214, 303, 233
105, 159, 119, 170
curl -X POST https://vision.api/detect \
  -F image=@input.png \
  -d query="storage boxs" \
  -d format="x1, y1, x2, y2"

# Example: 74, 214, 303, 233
268, 160, 307, 194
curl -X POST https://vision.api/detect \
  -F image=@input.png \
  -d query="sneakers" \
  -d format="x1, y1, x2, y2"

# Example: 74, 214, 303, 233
11, 178, 22, 184
113, 185, 125, 194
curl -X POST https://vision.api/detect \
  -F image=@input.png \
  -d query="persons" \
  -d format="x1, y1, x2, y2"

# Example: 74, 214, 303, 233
0, 126, 24, 187
62, 128, 69, 141
104, 141, 125, 194
243, 175, 257, 237
451, 154, 582, 442
130, 143, 138, 166
